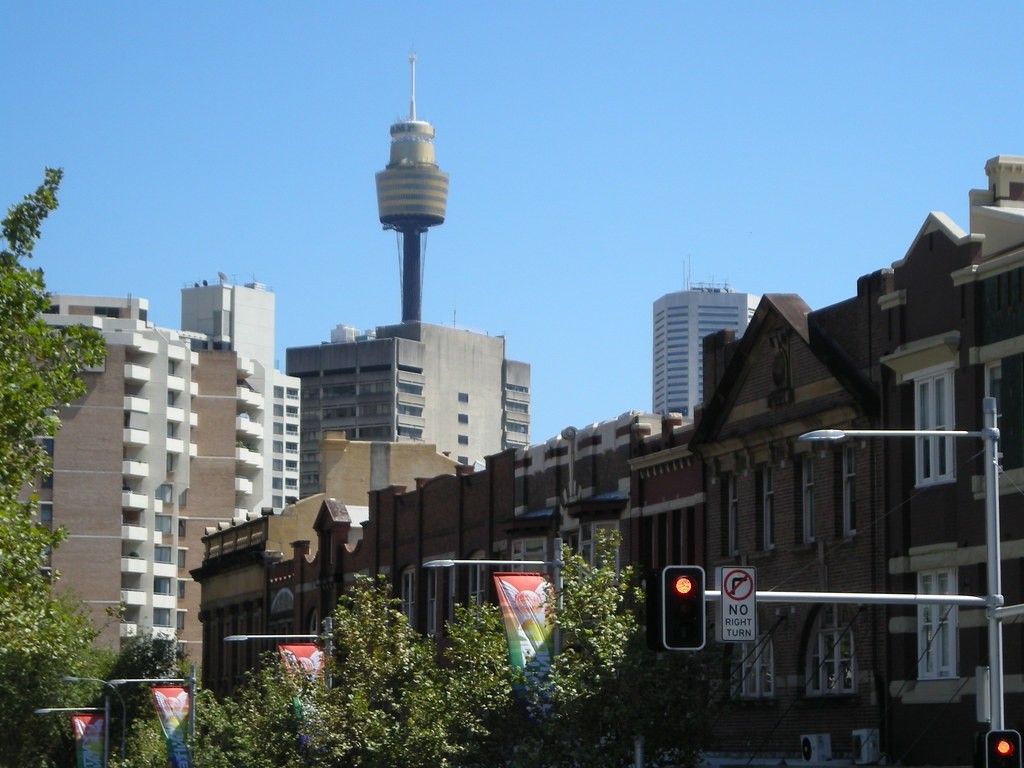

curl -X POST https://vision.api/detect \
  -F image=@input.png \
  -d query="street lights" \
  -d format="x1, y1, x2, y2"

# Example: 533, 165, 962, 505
62, 676, 125, 765
423, 558, 565, 664
35, 706, 110, 768
224, 631, 333, 690
798, 426, 1005, 729
110, 674, 196, 768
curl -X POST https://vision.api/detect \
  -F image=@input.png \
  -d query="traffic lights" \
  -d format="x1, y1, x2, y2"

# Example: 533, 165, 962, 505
643, 565, 707, 652
975, 730, 1022, 768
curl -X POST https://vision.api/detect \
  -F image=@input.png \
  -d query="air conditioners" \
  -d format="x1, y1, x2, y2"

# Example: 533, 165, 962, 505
801, 733, 831, 762
852, 729, 880, 765
